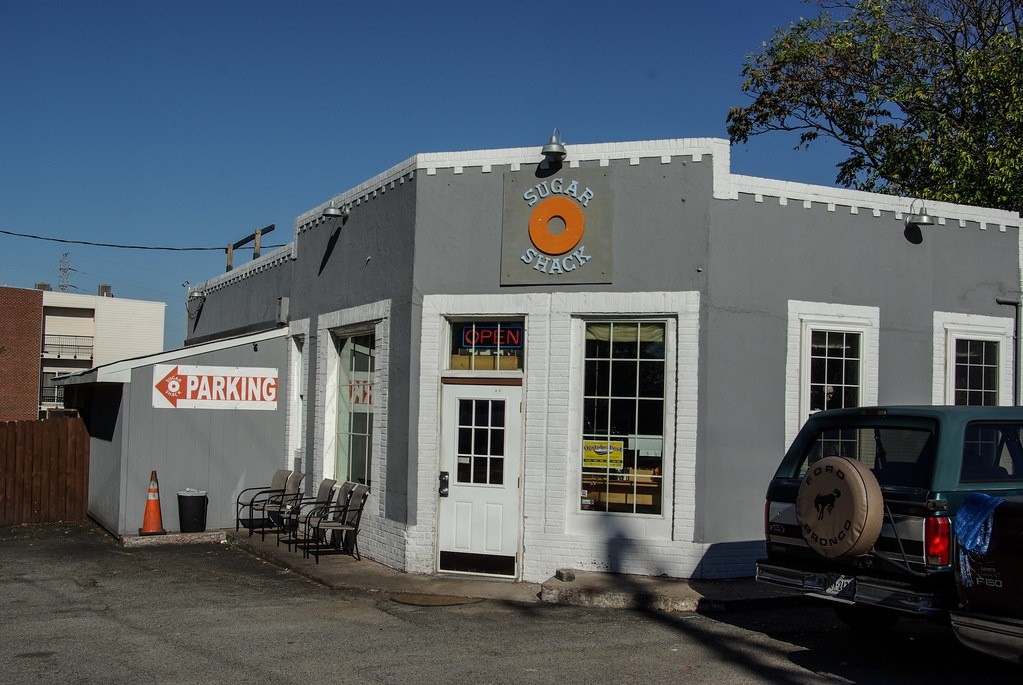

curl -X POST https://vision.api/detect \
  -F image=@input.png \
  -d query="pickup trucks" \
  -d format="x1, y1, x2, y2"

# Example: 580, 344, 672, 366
949, 494, 1022, 661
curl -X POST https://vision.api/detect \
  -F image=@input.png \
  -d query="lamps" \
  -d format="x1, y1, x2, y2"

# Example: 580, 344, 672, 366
905, 197, 934, 225
541, 127, 566, 156
189, 283, 206, 298
322, 193, 350, 217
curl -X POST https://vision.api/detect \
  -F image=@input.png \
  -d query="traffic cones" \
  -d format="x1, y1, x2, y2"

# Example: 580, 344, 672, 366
139, 471, 167, 536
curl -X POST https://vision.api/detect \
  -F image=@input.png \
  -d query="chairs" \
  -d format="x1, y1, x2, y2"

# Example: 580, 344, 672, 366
236, 469, 292, 535
277, 477, 338, 552
306, 484, 371, 565
295, 482, 356, 558
248, 471, 305, 542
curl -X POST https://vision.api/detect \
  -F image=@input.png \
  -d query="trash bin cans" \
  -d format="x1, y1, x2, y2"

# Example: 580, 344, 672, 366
176, 491, 209, 532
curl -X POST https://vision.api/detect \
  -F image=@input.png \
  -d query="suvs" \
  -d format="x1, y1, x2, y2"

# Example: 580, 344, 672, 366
755, 404, 1022, 617
